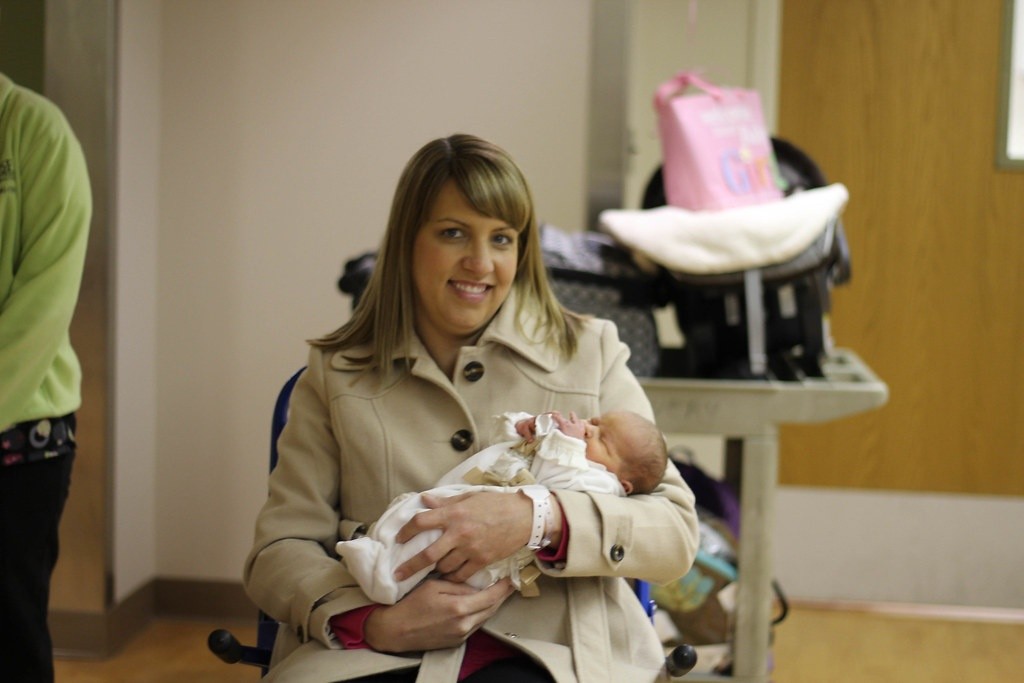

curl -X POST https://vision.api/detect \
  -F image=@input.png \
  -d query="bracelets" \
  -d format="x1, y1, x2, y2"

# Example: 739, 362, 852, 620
518, 487, 551, 550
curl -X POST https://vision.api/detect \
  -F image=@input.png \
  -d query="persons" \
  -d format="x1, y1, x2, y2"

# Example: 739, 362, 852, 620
241, 134, 700, 683
0, 72, 92, 683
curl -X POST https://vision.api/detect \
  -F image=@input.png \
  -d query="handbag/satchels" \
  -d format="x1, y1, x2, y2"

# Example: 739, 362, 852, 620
536, 225, 658, 377
653, 72, 784, 212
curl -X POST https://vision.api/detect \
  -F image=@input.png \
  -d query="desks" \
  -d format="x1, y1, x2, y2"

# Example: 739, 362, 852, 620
634, 345, 888, 683
